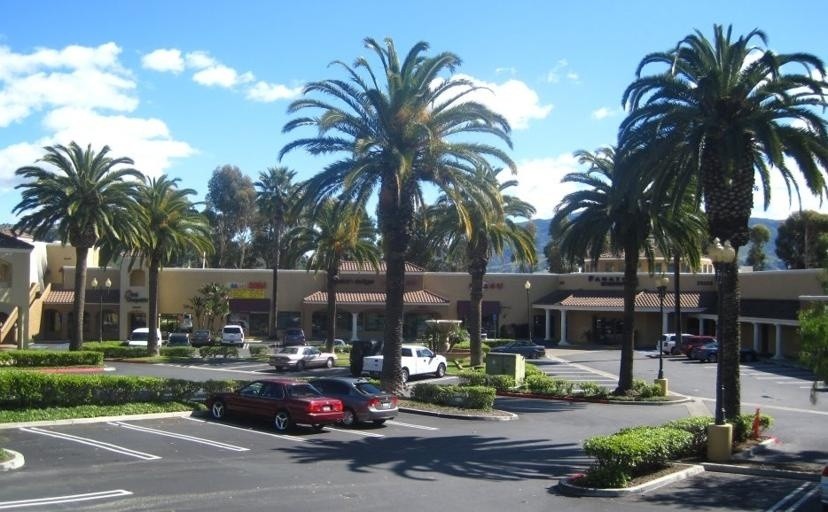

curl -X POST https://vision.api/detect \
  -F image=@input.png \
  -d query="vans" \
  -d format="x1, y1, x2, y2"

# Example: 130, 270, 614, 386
125, 327, 162, 347
282, 327, 305, 346
220, 325, 246, 346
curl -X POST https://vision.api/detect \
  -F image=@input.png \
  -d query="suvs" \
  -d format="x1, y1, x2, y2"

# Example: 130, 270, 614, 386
682, 336, 717, 361
656, 333, 693, 355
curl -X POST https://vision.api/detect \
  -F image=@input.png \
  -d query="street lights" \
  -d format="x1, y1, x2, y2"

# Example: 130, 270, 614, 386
522, 280, 535, 344
698, 235, 740, 426
90, 276, 112, 342
651, 272, 670, 378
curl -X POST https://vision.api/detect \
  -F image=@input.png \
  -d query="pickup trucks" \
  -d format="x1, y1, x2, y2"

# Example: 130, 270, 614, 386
361, 343, 447, 384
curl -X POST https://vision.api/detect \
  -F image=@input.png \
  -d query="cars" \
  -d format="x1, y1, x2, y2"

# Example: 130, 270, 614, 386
205, 379, 344, 432
189, 329, 214, 348
323, 337, 345, 346
302, 374, 398, 430
697, 339, 758, 363
479, 331, 490, 342
488, 340, 546, 359
266, 345, 338, 372
166, 333, 189, 346
